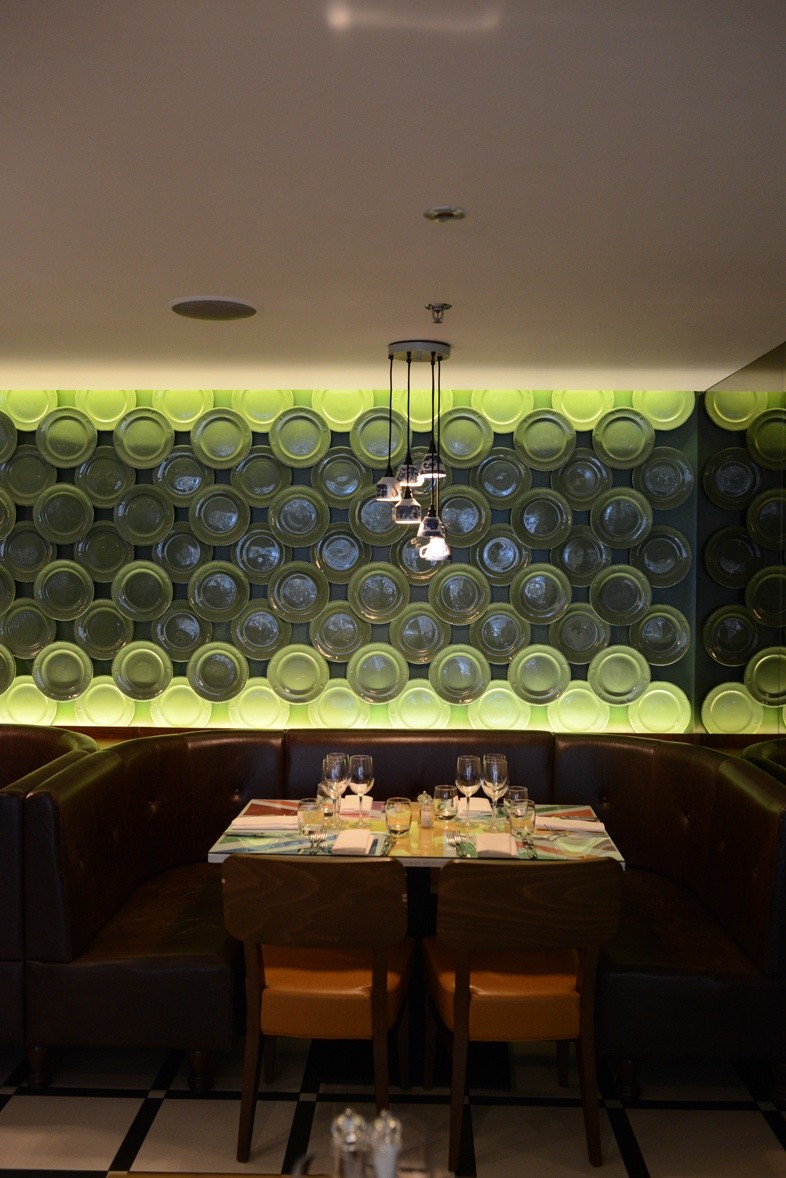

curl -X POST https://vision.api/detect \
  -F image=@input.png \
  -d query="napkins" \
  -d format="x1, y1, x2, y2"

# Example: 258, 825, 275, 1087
475, 832, 519, 858
535, 815, 605, 833
332, 829, 375, 855
340, 795, 373, 815
232, 813, 299, 832
459, 797, 493, 815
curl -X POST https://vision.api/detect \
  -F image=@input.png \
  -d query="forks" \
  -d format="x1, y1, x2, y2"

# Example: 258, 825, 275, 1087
446, 830, 466, 858
308, 829, 327, 856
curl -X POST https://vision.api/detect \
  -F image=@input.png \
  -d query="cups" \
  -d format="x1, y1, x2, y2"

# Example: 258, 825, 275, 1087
509, 800, 536, 836
502, 786, 530, 817
434, 784, 459, 821
298, 799, 323, 835
385, 798, 412, 837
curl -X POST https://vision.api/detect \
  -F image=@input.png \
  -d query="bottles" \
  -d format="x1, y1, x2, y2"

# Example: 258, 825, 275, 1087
332, 1102, 402, 1178
418, 791, 436, 829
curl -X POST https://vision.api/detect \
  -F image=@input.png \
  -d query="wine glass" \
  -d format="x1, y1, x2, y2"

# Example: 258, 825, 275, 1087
481, 752, 507, 833
321, 758, 348, 828
325, 751, 351, 813
456, 753, 482, 829
348, 754, 375, 828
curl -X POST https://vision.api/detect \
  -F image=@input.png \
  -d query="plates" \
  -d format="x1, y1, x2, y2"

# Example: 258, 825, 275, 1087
1, 383, 698, 737
704, 387, 786, 737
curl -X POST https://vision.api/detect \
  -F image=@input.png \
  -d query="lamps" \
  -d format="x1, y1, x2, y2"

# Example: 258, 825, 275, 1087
374, 339, 452, 562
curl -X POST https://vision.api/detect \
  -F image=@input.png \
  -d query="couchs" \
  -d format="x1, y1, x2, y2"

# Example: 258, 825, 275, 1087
0, 725, 786, 1098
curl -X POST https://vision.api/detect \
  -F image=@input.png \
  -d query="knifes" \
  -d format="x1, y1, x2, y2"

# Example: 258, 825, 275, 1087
380, 832, 399, 857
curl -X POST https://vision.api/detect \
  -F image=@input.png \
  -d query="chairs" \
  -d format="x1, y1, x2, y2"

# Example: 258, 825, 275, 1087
425, 858, 624, 1170
220, 851, 416, 1163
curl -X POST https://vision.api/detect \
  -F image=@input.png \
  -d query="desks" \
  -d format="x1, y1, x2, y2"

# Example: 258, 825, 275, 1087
207, 798, 627, 1091
